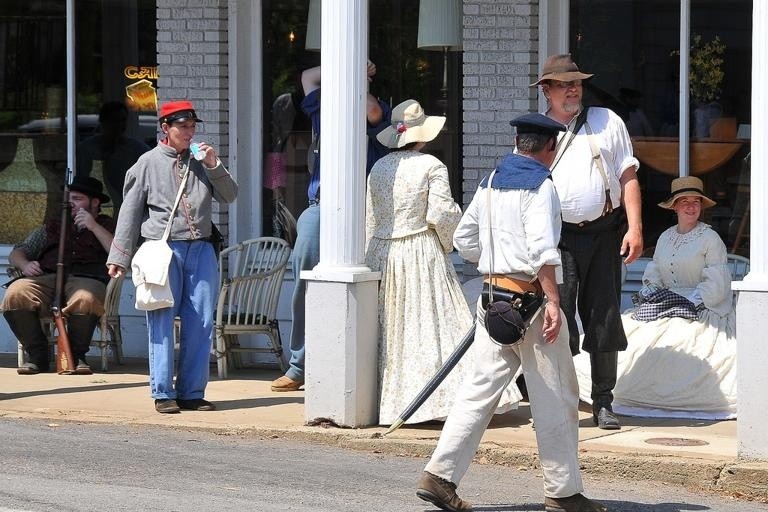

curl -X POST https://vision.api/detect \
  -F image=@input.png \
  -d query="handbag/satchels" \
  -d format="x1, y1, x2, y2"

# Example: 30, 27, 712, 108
480, 284, 535, 323
131, 239, 175, 311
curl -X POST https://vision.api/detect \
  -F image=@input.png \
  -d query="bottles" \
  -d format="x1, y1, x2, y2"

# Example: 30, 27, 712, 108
187, 137, 208, 160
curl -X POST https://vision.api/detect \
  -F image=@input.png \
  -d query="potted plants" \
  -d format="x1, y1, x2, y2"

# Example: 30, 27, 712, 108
676, 33, 722, 106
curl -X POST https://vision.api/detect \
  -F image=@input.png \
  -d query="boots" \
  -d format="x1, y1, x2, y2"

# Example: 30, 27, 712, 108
65, 313, 100, 376
1, 308, 54, 375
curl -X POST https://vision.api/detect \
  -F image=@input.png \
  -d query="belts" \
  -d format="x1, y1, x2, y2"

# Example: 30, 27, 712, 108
483, 273, 539, 294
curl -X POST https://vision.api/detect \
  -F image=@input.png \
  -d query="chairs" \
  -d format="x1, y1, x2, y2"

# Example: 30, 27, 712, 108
17, 262, 127, 371
727, 255, 751, 307
172, 237, 292, 380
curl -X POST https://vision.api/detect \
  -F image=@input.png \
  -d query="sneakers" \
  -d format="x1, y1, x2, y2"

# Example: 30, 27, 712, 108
591, 400, 622, 431
414, 469, 475, 512
543, 492, 618, 512
269, 375, 306, 393
154, 399, 183, 414
176, 397, 217, 412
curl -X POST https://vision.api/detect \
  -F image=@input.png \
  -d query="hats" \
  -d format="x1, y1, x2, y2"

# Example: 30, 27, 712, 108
527, 52, 596, 87
507, 111, 568, 137
375, 97, 448, 149
656, 174, 719, 211
158, 100, 202, 128
67, 172, 112, 206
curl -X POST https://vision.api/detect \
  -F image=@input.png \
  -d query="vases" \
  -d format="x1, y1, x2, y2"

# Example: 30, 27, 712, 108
690, 99, 721, 140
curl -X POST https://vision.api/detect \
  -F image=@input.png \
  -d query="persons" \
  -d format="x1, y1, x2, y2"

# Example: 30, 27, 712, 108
366, 99, 522, 426
272, 61, 391, 391
416, 112, 610, 512
570, 177, 737, 420
105, 100, 238, 413
512, 53, 644, 430
0, 175, 116, 376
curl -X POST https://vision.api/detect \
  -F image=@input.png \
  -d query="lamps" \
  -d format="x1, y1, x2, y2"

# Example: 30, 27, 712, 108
304, 0, 321, 53
417, 0, 464, 131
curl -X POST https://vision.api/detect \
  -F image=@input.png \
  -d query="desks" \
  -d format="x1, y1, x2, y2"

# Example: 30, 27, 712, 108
624, 133, 739, 180
